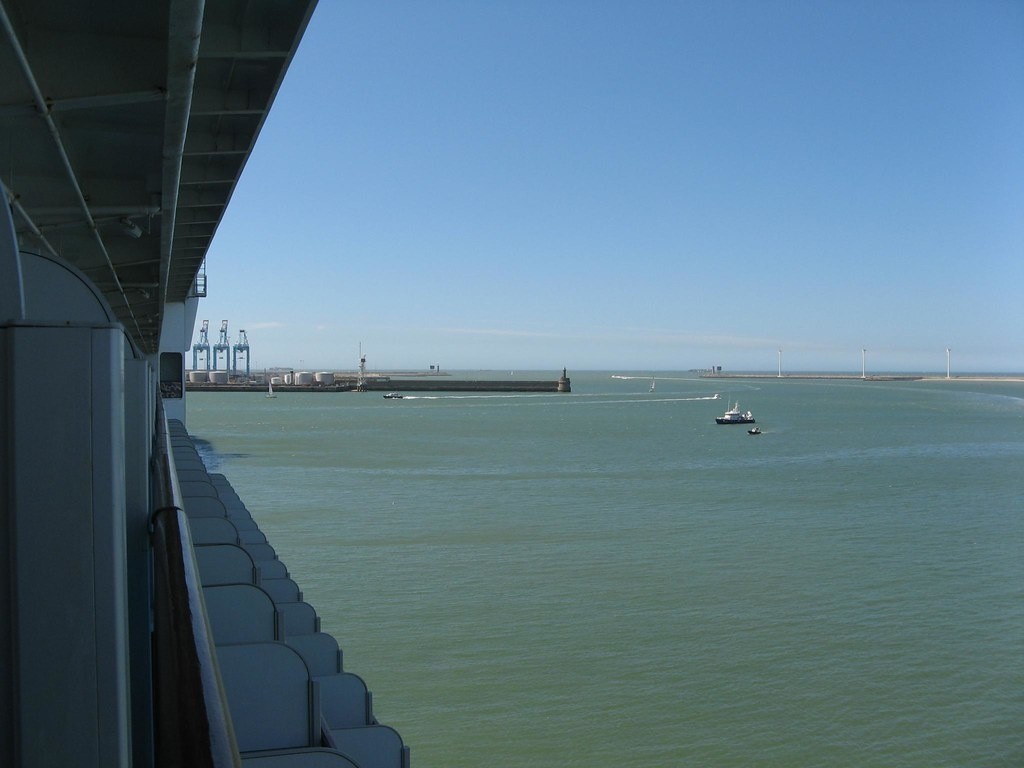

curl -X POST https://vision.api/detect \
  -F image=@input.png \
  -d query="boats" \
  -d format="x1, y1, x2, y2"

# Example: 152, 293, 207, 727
747, 426, 761, 435
714, 394, 756, 424
382, 392, 403, 399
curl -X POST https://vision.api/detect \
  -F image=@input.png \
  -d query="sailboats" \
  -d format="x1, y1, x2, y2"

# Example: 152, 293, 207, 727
264, 380, 277, 398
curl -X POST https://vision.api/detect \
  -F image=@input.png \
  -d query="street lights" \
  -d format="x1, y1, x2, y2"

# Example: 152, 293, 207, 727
861, 349, 866, 377
946, 349, 952, 377
778, 349, 782, 376
